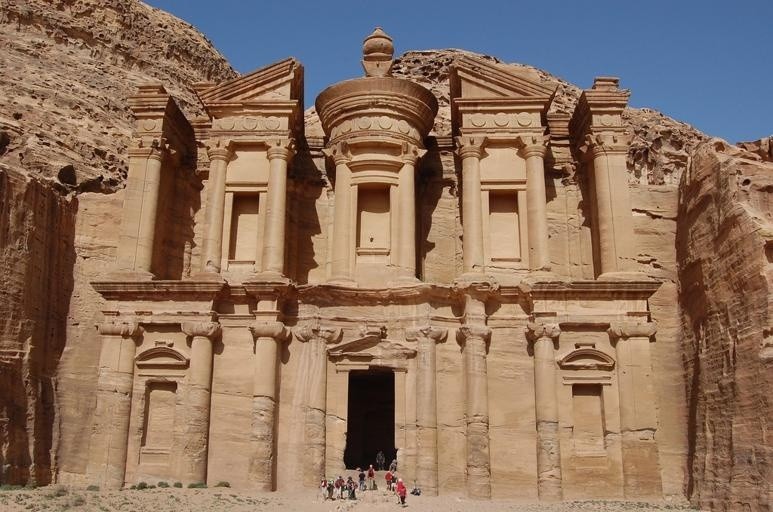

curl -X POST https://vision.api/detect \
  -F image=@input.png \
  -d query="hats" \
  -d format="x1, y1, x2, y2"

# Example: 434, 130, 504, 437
399, 477, 402, 482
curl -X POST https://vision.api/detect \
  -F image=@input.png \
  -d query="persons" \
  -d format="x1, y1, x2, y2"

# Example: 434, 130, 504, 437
384, 469, 392, 491
319, 476, 328, 500
357, 469, 364, 491
367, 463, 375, 491
397, 481, 407, 504
390, 472, 397, 491
346, 476, 356, 500
326, 478, 334, 499
334, 475, 344, 499
375, 450, 386, 470
393, 478, 401, 504
